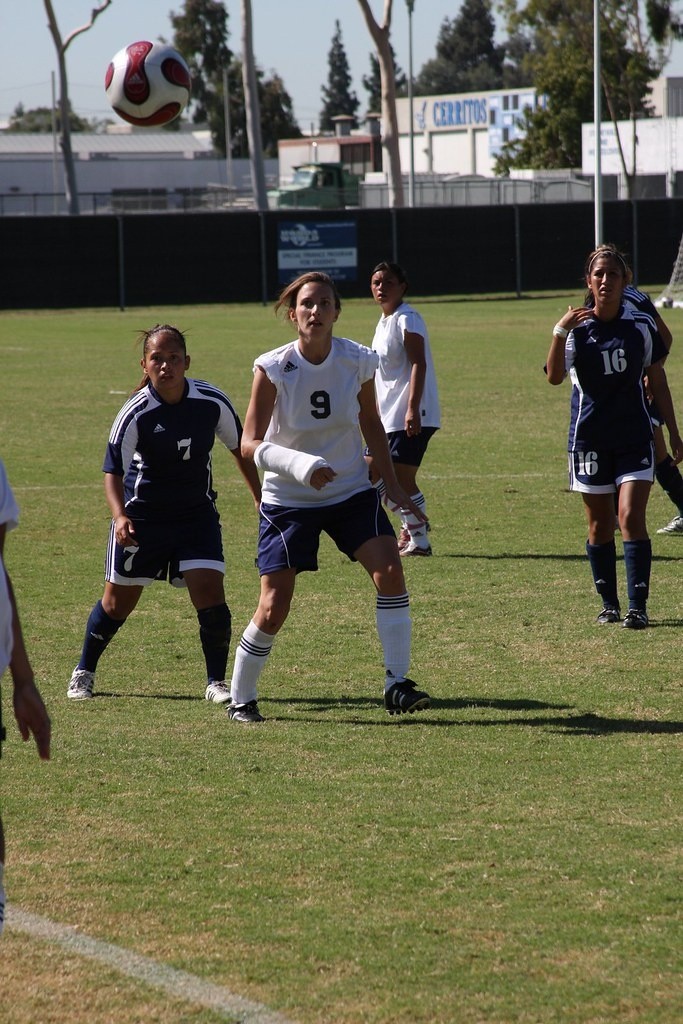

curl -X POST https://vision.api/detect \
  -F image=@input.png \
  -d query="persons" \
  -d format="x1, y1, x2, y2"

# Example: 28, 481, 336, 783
66, 323, 261, 703
227, 271, 429, 723
0, 456, 52, 918
585, 245, 683, 533
364, 262, 440, 558
543, 248, 683, 631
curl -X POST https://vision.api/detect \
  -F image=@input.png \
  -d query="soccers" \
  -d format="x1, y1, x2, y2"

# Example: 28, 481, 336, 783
106, 38, 189, 131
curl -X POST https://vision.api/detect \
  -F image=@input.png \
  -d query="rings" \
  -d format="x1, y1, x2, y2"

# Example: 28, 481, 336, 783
409, 426, 414, 428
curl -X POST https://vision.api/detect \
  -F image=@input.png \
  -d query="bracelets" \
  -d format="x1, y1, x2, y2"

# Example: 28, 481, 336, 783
552, 326, 569, 338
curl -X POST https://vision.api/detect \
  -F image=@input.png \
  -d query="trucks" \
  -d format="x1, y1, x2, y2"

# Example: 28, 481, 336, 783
274, 160, 594, 208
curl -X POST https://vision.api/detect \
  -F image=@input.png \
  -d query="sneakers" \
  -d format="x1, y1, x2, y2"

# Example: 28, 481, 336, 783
66, 664, 94, 701
597, 604, 621, 625
205, 679, 231, 704
396, 520, 431, 549
227, 699, 263, 723
657, 515, 683, 534
382, 669, 430, 715
622, 608, 649, 629
399, 540, 433, 557
